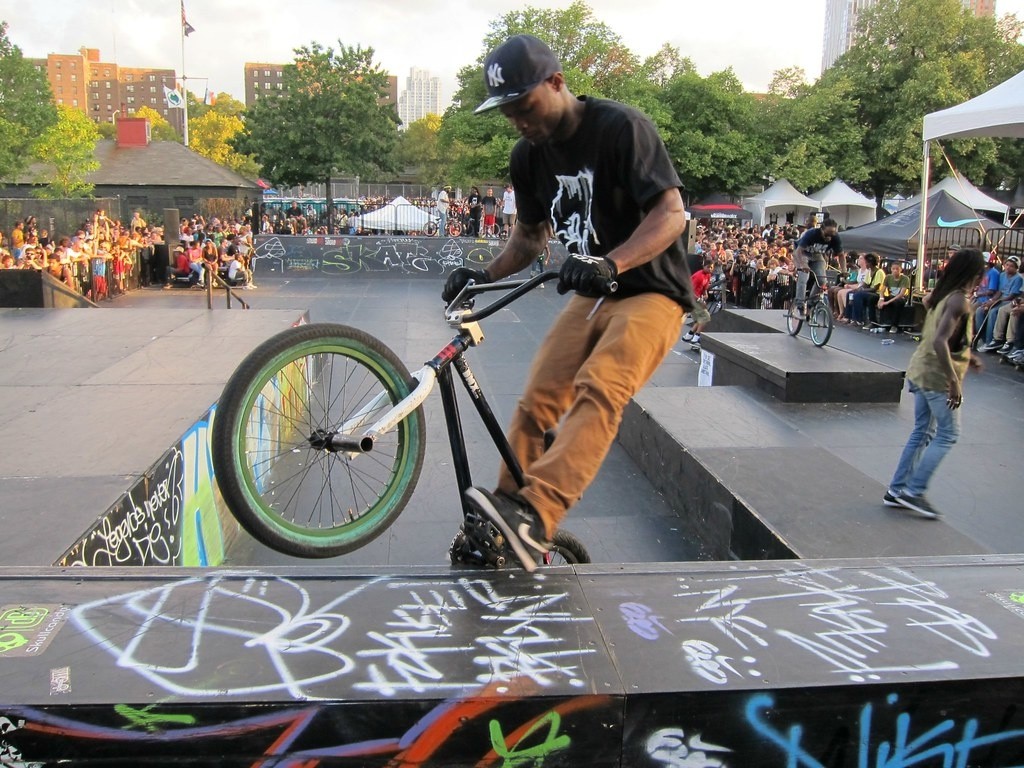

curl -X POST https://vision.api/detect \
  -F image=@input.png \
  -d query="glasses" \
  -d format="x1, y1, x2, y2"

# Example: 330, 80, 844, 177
823, 229, 837, 239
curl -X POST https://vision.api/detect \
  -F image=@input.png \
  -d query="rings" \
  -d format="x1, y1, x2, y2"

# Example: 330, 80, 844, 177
955, 402, 959, 405
947, 399, 951, 401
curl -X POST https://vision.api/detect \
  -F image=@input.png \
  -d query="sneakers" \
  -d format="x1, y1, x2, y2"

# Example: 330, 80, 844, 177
465, 487, 553, 572
883, 491, 908, 508
793, 305, 806, 320
895, 490, 938, 518
1013, 353, 1024, 361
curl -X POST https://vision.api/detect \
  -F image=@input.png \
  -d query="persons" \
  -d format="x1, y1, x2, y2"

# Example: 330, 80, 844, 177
791, 219, 850, 320
881, 247, 985, 519
695, 212, 1024, 370
682, 260, 714, 345
442, 34, 696, 571
0, 184, 559, 302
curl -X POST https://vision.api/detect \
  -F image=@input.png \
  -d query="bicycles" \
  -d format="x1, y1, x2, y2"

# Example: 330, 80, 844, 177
783, 268, 851, 347
423, 202, 462, 238
208, 266, 620, 567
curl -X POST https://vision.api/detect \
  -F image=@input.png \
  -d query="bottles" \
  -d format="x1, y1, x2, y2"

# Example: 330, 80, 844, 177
881, 339, 895, 344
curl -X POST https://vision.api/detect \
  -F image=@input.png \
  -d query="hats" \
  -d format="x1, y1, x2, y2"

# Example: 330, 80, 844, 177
173, 246, 184, 253
981, 251, 996, 263
948, 244, 962, 251
1007, 256, 1021, 269
444, 184, 451, 189
474, 35, 563, 115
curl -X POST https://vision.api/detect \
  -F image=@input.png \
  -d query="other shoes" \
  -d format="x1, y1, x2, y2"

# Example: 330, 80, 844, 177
247, 283, 258, 288
541, 282, 544, 288
691, 335, 700, 343
997, 342, 1016, 353
976, 343, 990, 353
685, 333, 693, 340
984, 339, 1004, 349
242, 286, 253, 291
833, 312, 898, 333
1006, 347, 1024, 359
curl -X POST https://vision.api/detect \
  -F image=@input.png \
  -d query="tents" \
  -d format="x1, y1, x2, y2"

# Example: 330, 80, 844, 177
915, 68, 1024, 293
687, 171, 1024, 272
347, 195, 439, 232
256, 180, 278, 195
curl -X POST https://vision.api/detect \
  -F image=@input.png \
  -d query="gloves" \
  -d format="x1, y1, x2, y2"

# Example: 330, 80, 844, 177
441, 268, 487, 301
557, 253, 617, 295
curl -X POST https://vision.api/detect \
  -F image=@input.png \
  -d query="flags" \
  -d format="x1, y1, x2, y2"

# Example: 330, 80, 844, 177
205, 88, 217, 106
164, 86, 184, 109
182, 7, 194, 37
